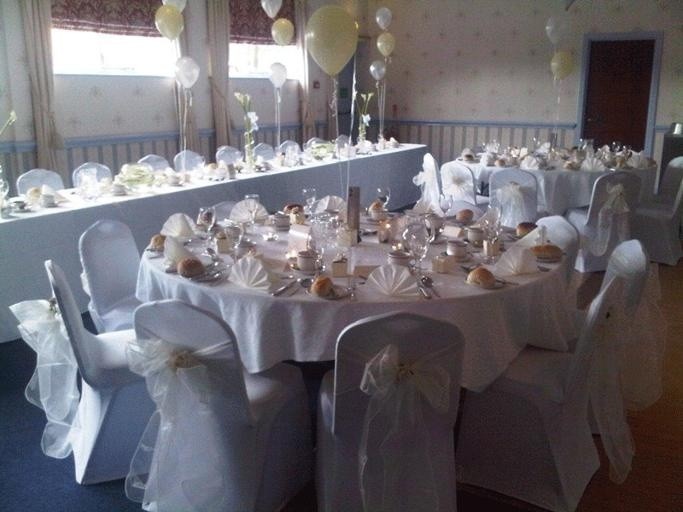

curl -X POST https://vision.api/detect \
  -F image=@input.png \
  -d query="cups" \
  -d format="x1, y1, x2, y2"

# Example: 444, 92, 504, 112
168, 177, 180, 184
113, 184, 123, 193
13, 201, 25, 209
39, 194, 54, 204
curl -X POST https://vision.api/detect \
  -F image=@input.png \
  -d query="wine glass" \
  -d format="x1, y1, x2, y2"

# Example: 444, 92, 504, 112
197, 186, 500, 275
457, 135, 631, 166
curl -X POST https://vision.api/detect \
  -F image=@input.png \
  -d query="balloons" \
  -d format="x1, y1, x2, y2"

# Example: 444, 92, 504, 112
551, 52, 573, 80
546, 12, 571, 46
155, 1, 396, 89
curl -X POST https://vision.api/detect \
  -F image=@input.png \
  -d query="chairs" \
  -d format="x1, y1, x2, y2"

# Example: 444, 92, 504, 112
1, 130, 429, 228
318, 308, 463, 512
422, 144, 683, 285
76, 217, 141, 332
461, 273, 618, 512
124, 299, 311, 512
567, 238, 648, 483
5, 259, 156, 484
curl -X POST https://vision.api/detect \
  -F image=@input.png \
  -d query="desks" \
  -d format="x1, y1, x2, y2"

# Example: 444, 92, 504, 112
142, 210, 571, 307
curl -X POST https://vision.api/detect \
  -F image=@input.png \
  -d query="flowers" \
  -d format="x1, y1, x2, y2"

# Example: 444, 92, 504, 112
231, 92, 259, 136
357, 92, 375, 129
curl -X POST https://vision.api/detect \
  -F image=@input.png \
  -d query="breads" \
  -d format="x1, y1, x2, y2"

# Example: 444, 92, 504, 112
151, 235, 163, 247
516, 222, 538, 237
467, 268, 496, 287
456, 209, 473, 222
177, 257, 203, 275
369, 200, 383, 210
282, 204, 304, 215
530, 244, 562, 262
311, 276, 332, 297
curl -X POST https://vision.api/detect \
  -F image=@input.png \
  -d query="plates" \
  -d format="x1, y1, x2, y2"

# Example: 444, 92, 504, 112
43, 203, 57, 208
169, 184, 181, 187
144, 235, 560, 297
113, 192, 126, 196
14, 208, 30, 211
